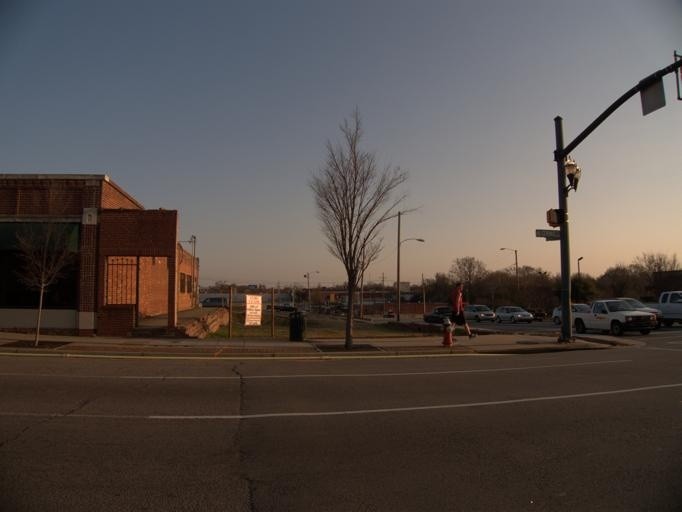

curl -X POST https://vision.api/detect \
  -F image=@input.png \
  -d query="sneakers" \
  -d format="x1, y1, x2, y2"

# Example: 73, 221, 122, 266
468, 333, 478, 339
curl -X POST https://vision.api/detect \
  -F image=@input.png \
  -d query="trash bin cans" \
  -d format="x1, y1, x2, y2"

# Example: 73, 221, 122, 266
289, 313, 306, 341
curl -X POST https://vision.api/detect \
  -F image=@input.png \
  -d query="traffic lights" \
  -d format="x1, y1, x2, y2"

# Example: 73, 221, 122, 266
546, 209, 560, 228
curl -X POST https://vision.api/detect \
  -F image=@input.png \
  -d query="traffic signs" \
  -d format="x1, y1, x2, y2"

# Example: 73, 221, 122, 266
536, 229, 561, 241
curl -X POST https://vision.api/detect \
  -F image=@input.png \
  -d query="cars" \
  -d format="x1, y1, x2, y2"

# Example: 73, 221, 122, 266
464, 305, 546, 324
552, 304, 591, 325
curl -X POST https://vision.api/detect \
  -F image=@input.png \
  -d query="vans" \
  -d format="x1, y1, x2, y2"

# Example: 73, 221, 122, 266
200, 296, 229, 309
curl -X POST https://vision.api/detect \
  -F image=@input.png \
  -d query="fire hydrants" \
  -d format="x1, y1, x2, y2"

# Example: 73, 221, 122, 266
440, 317, 455, 347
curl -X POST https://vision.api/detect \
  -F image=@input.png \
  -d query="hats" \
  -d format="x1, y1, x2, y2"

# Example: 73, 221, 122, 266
455, 279, 465, 284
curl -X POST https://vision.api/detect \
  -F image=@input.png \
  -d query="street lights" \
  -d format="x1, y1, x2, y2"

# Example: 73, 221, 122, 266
306, 270, 320, 311
556, 157, 581, 343
397, 238, 425, 321
500, 248, 520, 295
578, 257, 583, 277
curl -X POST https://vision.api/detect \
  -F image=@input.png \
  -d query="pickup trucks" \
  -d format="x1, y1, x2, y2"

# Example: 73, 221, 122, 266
267, 303, 298, 312
423, 307, 452, 323
572, 290, 682, 336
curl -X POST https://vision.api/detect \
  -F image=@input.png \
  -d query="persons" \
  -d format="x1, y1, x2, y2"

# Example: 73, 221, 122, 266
448, 282, 479, 338
